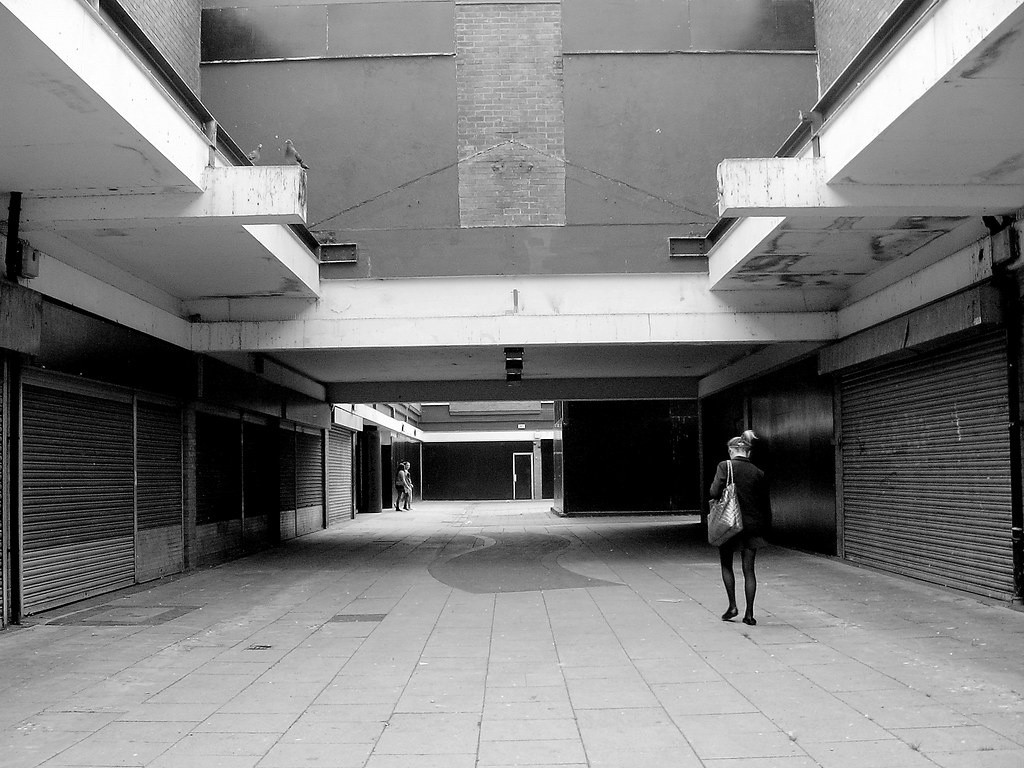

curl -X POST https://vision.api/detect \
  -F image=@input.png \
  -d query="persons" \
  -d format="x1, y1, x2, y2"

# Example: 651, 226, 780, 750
395, 461, 414, 511
709, 430, 772, 625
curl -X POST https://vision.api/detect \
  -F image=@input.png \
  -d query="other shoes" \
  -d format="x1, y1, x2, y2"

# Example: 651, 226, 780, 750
396, 507, 400, 511
403, 507, 408, 511
743, 618, 756, 625
722, 607, 738, 619
409, 507, 412, 510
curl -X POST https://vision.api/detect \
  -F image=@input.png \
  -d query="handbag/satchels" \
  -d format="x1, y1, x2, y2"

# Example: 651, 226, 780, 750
707, 459, 744, 547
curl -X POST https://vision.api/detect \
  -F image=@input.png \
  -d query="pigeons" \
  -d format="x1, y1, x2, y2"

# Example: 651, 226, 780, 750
284, 140, 310, 170
246, 144, 262, 163
